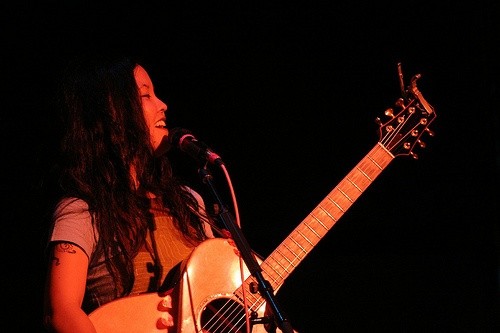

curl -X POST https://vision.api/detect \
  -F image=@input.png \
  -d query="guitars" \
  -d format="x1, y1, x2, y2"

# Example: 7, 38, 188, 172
87, 61, 438, 333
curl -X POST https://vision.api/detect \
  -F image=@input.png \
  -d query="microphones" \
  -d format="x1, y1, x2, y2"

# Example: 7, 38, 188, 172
180, 134, 225, 167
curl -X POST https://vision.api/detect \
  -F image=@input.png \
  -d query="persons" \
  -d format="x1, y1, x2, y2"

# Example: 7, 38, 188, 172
42, 61, 216, 333
